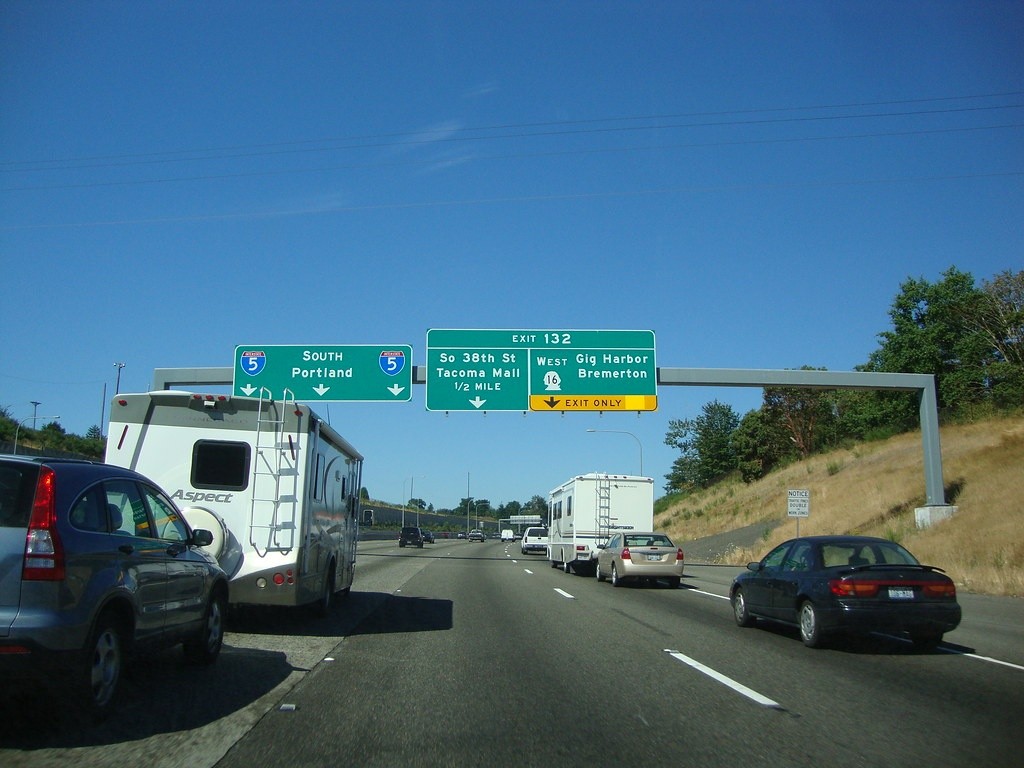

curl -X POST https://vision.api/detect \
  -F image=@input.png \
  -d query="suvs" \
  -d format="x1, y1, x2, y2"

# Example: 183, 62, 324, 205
397, 527, 425, 548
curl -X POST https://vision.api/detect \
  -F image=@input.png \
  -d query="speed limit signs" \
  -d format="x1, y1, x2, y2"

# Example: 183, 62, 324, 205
379, 349, 406, 377
241, 351, 266, 376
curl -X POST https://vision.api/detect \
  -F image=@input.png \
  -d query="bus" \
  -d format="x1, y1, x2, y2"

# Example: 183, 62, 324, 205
542, 472, 654, 572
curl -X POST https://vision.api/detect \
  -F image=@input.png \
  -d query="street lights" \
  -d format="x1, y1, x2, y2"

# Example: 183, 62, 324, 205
30, 400, 43, 431
401, 474, 427, 528
468, 498, 478, 534
13, 415, 60, 455
586, 428, 643, 477
475, 502, 490, 530
113, 361, 127, 394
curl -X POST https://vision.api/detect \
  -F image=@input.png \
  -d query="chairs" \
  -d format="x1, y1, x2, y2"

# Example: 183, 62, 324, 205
848, 558, 869, 565
647, 541, 653, 545
84, 503, 132, 536
653, 541, 664, 545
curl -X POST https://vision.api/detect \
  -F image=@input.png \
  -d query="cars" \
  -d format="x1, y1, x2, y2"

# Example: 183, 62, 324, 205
420, 529, 435, 543
597, 532, 685, 587
0, 446, 233, 720
520, 526, 549, 555
728, 534, 962, 648
500, 529, 516, 543
468, 529, 485, 542
458, 531, 467, 540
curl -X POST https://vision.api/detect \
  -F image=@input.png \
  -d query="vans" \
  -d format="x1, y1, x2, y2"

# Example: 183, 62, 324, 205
94, 389, 366, 626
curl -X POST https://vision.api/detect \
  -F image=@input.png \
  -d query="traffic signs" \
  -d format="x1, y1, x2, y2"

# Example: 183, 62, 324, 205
424, 328, 660, 412
232, 344, 414, 403
